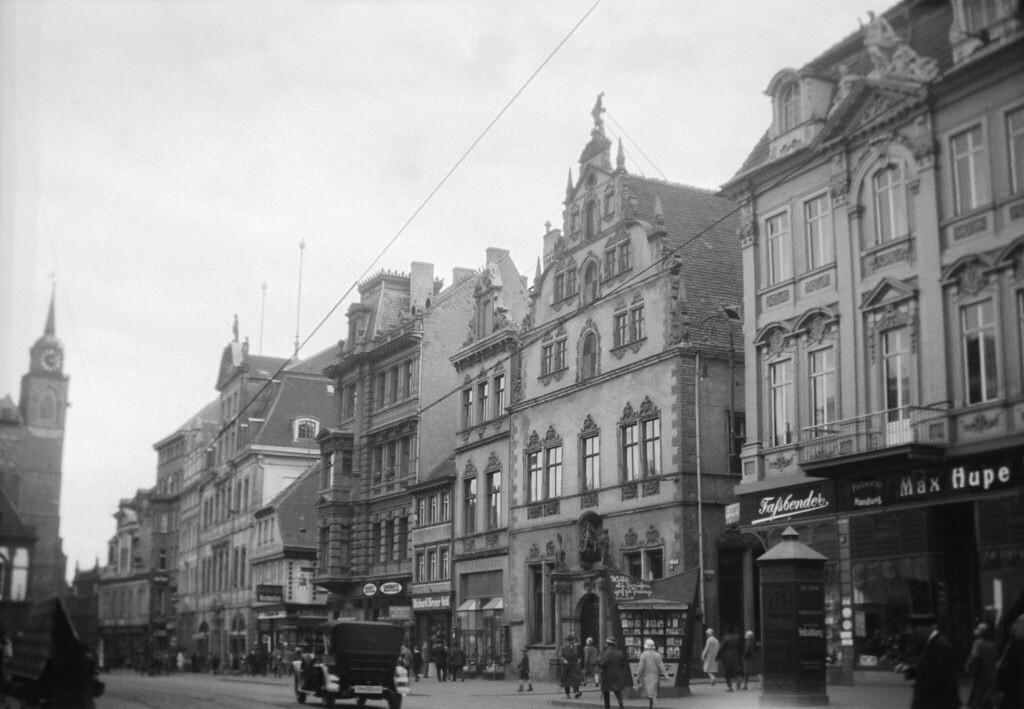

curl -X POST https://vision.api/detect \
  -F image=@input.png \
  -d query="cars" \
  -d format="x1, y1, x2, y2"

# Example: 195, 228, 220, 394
289, 617, 405, 709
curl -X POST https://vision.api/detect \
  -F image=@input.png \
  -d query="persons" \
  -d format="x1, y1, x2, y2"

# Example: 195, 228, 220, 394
580, 637, 601, 687
635, 638, 671, 708
597, 636, 634, 709
514, 649, 533, 692
701, 628, 721, 685
433, 639, 449, 682
715, 627, 756, 692
447, 642, 465, 682
894, 615, 1024, 708
399, 643, 424, 682
153, 642, 292, 679
557, 635, 584, 698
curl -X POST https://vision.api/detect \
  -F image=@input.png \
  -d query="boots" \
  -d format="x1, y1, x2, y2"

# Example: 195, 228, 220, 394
527, 683, 533, 691
516, 684, 524, 693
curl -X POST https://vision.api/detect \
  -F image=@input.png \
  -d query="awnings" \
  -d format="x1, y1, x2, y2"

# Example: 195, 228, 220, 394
618, 597, 689, 611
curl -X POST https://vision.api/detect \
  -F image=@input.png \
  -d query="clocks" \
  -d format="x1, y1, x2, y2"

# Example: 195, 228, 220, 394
41, 349, 62, 372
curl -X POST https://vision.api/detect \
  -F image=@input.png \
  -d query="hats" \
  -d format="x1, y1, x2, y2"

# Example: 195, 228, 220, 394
974, 623, 988, 634
565, 634, 573, 640
643, 638, 655, 649
745, 630, 754, 639
605, 636, 618, 645
705, 628, 714, 635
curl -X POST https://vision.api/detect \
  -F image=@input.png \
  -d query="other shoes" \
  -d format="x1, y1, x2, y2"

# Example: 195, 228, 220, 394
726, 689, 734, 692
566, 695, 570, 699
582, 683, 586, 686
711, 678, 716, 685
575, 692, 583, 699
595, 683, 599, 686
